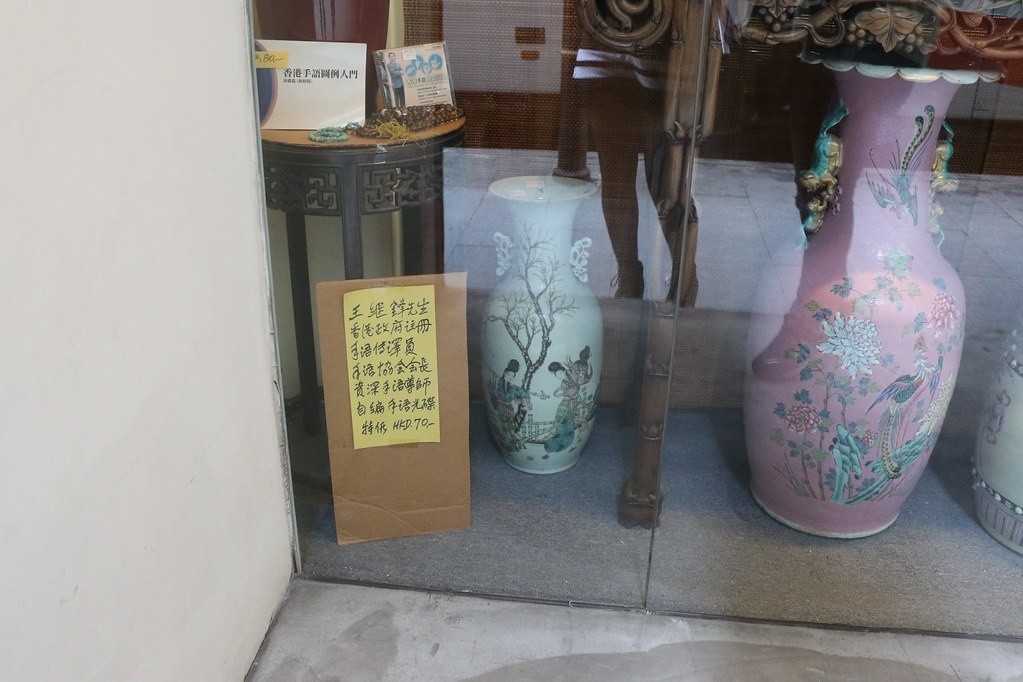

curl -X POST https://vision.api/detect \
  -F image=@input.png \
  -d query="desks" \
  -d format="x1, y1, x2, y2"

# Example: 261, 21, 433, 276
259, 106, 472, 486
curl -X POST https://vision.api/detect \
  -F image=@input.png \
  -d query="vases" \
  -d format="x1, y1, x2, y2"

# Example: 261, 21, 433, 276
743, 55, 1009, 539
972, 324, 1023, 556
480, 174, 603, 475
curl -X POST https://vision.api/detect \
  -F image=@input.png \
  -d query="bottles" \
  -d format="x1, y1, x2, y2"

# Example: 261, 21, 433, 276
743, 59, 1007, 539
480, 175, 604, 474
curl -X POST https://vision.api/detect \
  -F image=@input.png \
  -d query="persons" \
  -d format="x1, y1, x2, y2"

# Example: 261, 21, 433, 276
387, 52, 406, 107
581, 0, 700, 307
753, 0, 933, 383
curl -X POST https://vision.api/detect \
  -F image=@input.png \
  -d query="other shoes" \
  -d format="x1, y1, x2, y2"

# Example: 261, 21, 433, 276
610, 260, 644, 297
666, 269, 698, 309
